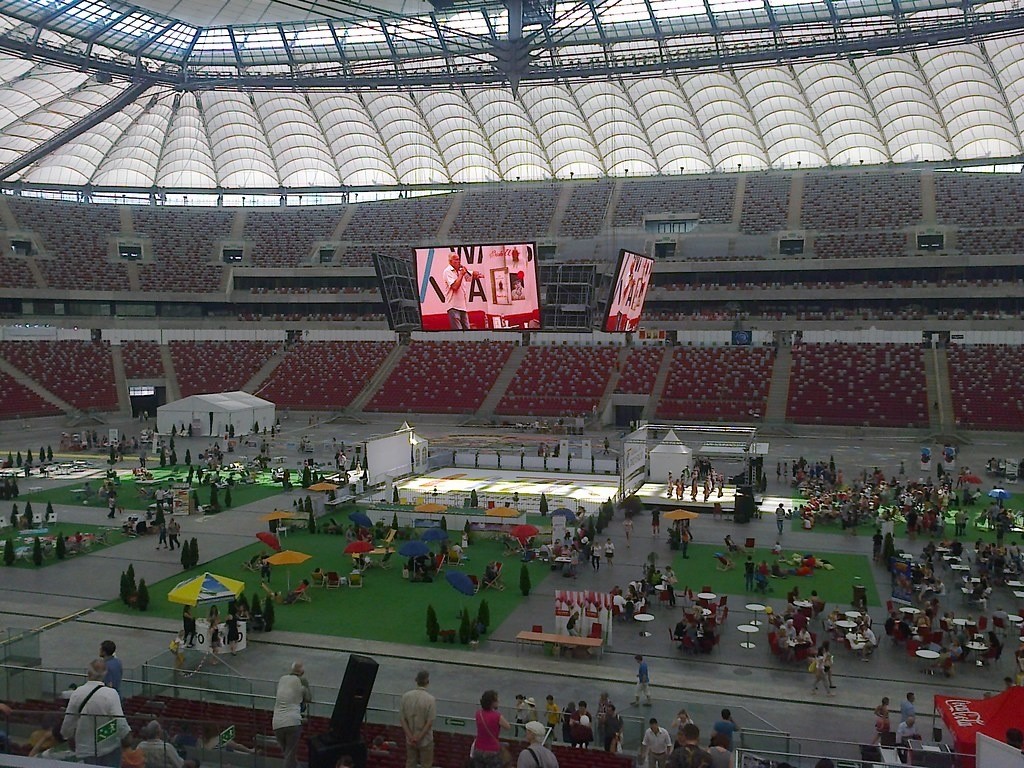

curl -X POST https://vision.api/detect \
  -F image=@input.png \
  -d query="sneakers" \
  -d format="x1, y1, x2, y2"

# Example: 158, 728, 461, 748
630, 702, 639, 707
642, 702, 652, 707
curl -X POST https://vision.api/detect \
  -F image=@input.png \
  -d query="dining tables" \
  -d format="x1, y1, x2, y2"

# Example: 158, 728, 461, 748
634, 544, 1024, 675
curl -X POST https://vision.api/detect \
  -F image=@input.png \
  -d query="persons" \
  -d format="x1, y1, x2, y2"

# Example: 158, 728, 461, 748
896, 716, 917, 745
195, 722, 257, 755
2, 407, 1023, 694
870, 697, 892, 744
671, 708, 693, 745
711, 708, 739, 743
59, 659, 134, 768
700, 732, 734, 768
56, 681, 77, 701
169, 722, 199, 747
630, 655, 652, 706
472, 688, 510, 768
612, 266, 636, 333
98, 638, 122, 692
30, 717, 86, 765
517, 722, 560, 768
641, 717, 671, 765
444, 253, 472, 330
899, 693, 916, 723
272, 660, 312, 767
135, 720, 200, 767
400, 670, 435, 767
512, 694, 626, 749
668, 723, 714, 767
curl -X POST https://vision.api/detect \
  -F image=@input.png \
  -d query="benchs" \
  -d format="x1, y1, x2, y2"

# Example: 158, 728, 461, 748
1, 693, 638, 768
0, 340, 1024, 436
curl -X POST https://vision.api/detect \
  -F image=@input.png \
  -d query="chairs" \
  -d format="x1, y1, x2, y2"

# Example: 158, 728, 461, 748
256, 517, 578, 604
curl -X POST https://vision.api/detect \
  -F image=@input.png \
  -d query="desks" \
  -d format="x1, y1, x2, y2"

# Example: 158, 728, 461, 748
515, 631, 603, 666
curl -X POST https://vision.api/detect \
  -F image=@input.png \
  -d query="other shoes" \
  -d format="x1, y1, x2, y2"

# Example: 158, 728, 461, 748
231, 652, 238, 656
810, 689, 820, 695
815, 686, 818, 690
826, 692, 836, 697
828, 685, 837, 688
860, 658, 869, 662
209, 658, 219, 666
666, 604, 677, 609
189, 642, 196, 646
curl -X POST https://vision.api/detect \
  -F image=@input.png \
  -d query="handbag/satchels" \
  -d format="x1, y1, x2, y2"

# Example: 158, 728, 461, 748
217, 635, 226, 648
590, 550, 594, 556
121, 746, 147, 767
168, 639, 179, 656
807, 660, 818, 673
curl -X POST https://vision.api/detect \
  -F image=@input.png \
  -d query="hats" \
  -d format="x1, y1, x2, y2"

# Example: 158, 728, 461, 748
524, 697, 536, 706
